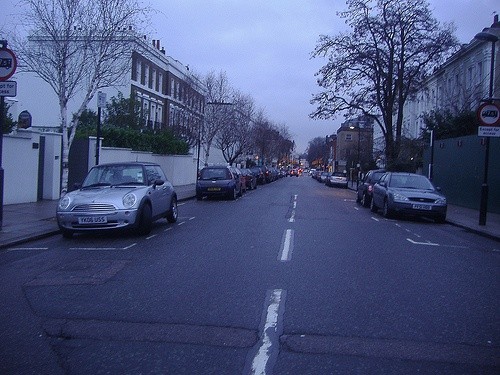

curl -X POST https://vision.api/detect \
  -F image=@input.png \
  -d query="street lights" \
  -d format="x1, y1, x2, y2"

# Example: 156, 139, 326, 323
350, 126, 361, 178
473, 31, 499, 224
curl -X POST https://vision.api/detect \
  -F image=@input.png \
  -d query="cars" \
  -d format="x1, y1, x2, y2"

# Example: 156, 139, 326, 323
308, 169, 348, 187
356, 169, 447, 222
198, 165, 307, 200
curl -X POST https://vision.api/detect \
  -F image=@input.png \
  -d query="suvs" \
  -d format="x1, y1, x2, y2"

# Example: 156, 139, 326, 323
56, 161, 178, 236
